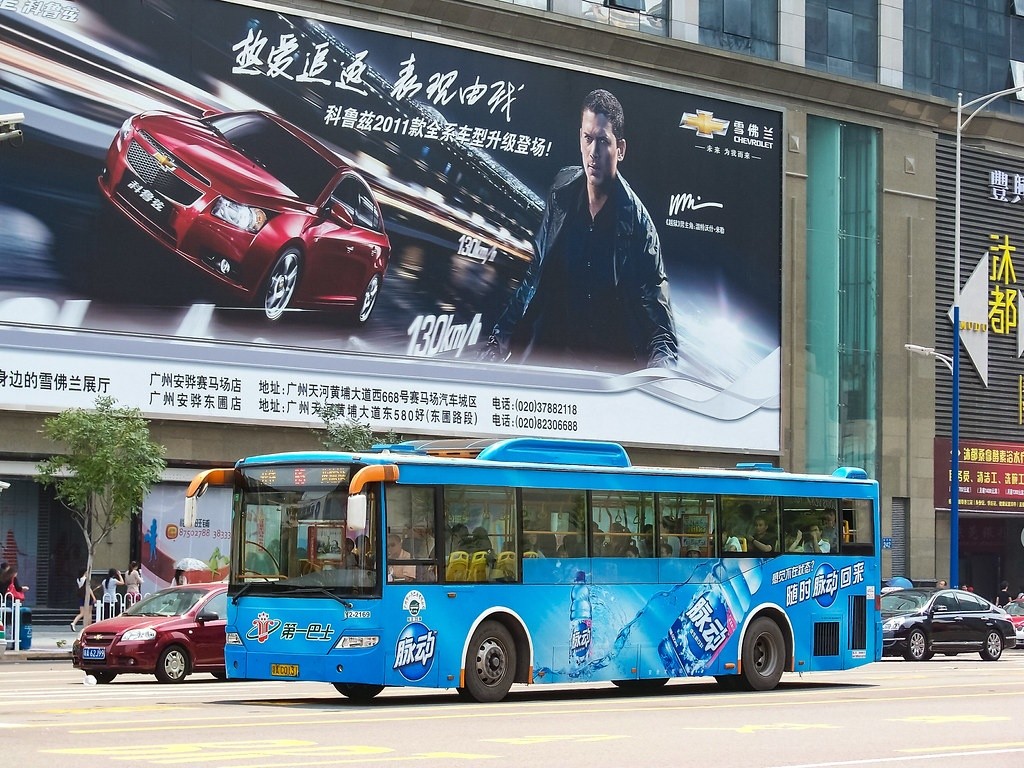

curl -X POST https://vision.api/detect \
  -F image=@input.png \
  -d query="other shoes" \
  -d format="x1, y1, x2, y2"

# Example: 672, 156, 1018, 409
71, 623, 76, 632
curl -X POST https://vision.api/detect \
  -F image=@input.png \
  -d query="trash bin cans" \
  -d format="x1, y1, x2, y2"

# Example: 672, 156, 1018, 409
0, 607, 13, 649
13, 608, 32, 651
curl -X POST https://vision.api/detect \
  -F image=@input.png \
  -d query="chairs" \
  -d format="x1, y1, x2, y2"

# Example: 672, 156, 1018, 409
444, 520, 836, 582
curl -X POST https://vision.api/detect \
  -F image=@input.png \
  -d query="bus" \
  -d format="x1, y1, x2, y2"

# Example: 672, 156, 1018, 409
185, 433, 887, 706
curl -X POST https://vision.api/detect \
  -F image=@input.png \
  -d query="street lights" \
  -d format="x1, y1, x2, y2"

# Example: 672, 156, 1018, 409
901, 81, 1023, 590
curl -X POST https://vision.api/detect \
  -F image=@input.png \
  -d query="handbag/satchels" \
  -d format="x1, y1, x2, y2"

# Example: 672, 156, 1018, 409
7, 577, 25, 600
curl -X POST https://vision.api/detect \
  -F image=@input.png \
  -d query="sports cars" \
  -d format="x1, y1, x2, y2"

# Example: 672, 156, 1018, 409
94, 107, 393, 327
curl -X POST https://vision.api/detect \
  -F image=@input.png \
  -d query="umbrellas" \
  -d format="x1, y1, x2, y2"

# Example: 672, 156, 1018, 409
172, 558, 208, 572
884, 577, 913, 588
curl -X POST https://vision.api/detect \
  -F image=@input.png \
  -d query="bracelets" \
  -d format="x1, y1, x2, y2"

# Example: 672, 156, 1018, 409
752, 539, 756, 542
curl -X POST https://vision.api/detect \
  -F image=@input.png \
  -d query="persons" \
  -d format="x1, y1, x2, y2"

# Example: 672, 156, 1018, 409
403, 524, 492, 583
712, 501, 846, 552
387, 535, 416, 583
962, 585, 973, 592
125, 561, 143, 609
345, 534, 374, 569
660, 515, 701, 558
317, 531, 341, 554
593, 520, 653, 557
0, 563, 29, 625
102, 569, 124, 619
939, 580, 947, 587
71, 568, 96, 632
522, 530, 545, 558
479, 88, 678, 372
996, 581, 1012, 608
170, 569, 187, 586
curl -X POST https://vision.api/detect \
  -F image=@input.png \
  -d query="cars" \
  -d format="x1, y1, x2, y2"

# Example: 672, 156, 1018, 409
69, 581, 229, 684
1002, 592, 1024, 650
879, 586, 1017, 663
